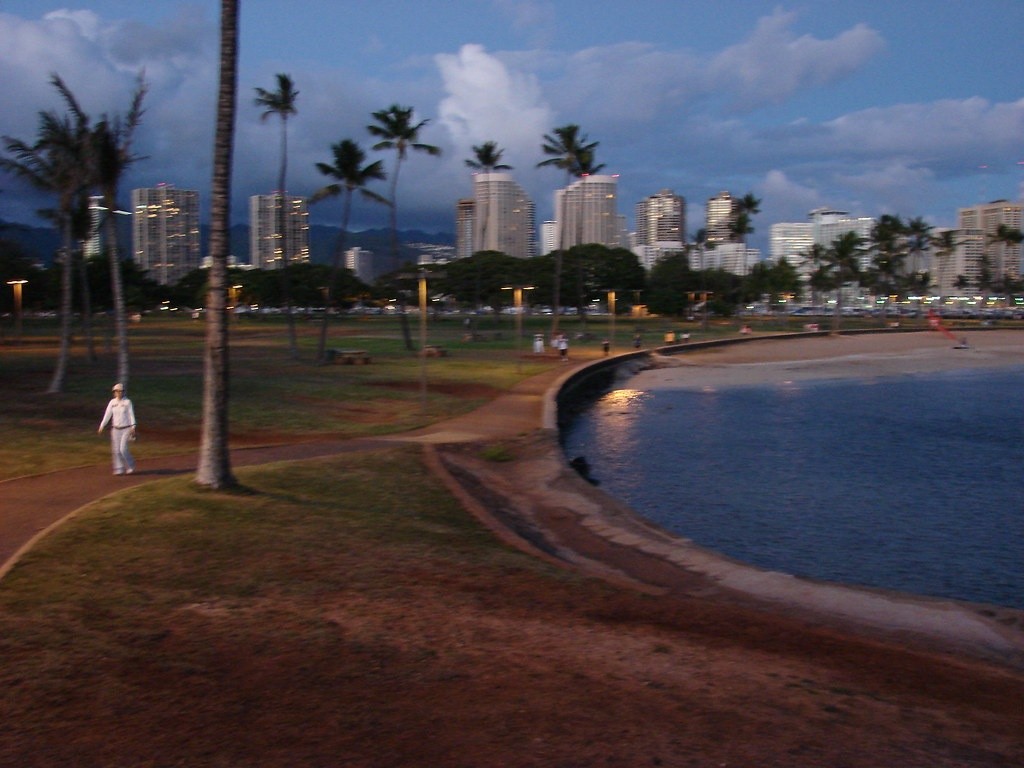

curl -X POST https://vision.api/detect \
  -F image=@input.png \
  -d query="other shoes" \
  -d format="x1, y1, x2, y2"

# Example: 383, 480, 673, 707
115, 469, 124, 474
127, 465, 136, 473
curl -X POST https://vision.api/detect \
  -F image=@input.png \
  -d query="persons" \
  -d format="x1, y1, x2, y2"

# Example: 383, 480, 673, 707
601, 337, 610, 357
665, 329, 690, 345
739, 324, 752, 336
632, 331, 642, 351
534, 329, 569, 362
98, 384, 138, 476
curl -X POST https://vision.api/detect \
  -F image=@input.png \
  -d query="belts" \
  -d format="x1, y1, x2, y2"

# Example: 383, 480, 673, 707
113, 425, 130, 429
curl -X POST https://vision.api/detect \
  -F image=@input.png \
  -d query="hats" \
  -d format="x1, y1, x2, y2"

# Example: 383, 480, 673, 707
113, 384, 124, 390
